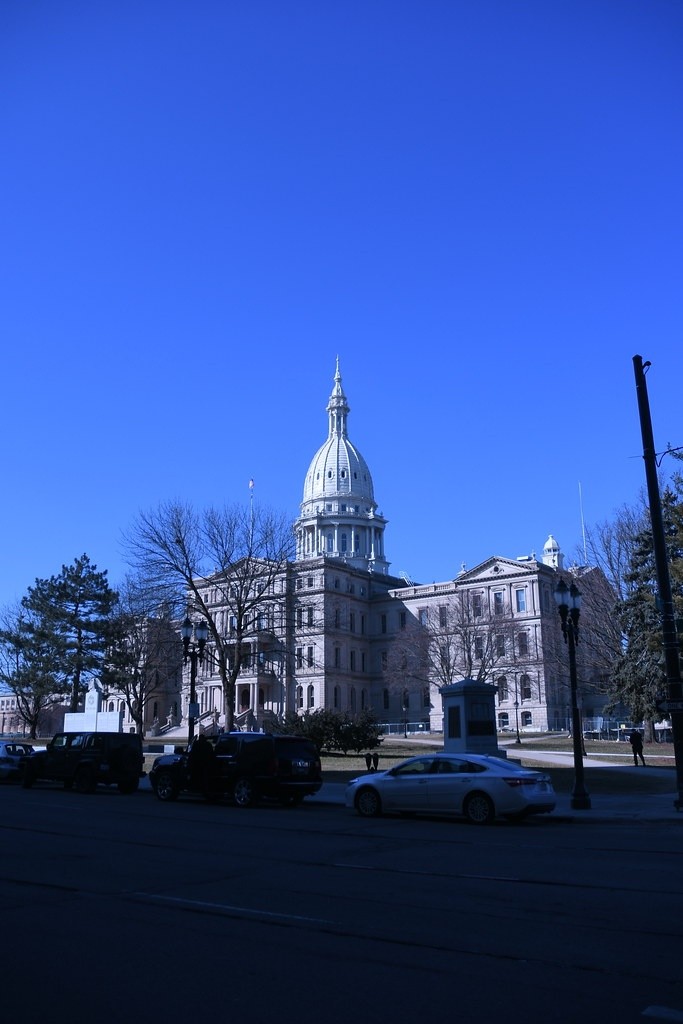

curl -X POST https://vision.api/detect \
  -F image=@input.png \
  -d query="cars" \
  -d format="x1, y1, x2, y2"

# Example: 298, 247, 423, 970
344, 752, 556, 825
0, 742, 36, 782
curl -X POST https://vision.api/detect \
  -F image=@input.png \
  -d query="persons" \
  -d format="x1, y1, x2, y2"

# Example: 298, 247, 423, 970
191, 733, 213, 786
629, 729, 646, 767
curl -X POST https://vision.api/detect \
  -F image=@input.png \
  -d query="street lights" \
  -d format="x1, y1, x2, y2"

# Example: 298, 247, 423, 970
514, 700, 522, 744
555, 576, 593, 810
180, 617, 208, 747
402, 706, 408, 738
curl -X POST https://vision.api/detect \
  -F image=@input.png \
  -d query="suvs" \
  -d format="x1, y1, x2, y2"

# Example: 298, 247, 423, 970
21, 731, 144, 793
147, 731, 324, 811
